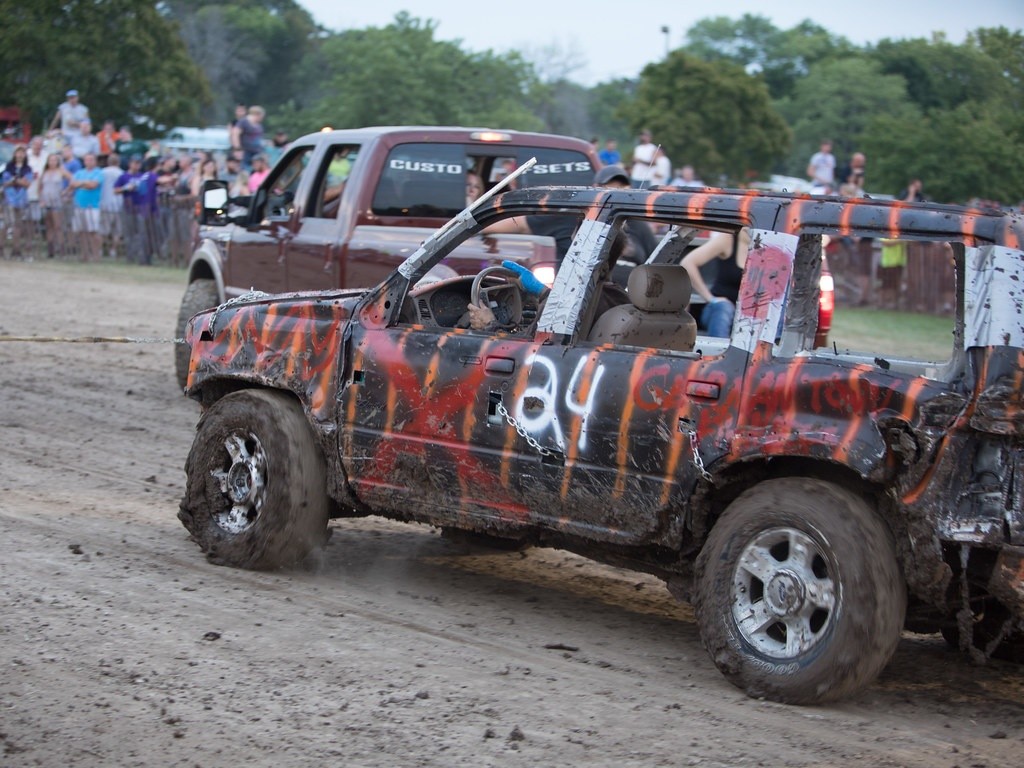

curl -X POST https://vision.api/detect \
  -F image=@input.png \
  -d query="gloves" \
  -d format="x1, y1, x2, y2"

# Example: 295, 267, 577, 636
501, 259, 547, 296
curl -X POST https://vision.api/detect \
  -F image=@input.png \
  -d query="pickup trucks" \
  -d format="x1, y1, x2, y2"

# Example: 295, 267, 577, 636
173, 125, 835, 393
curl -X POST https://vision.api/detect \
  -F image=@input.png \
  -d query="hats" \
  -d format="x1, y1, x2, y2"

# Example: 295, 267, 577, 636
593, 165, 632, 186
130, 154, 143, 161
66, 89, 79, 97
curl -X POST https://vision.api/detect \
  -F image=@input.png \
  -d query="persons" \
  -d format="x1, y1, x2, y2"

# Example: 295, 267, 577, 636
679, 225, 787, 344
0, 89, 354, 263
475, 166, 662, 289
589, 126, 707, 189
467, 219, 631, 340
807, 137, 926, 204
877, 238, 904, 310
465, 169, 482, 203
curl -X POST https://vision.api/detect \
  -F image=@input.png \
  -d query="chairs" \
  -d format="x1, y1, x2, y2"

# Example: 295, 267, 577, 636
589, 264, 699, 351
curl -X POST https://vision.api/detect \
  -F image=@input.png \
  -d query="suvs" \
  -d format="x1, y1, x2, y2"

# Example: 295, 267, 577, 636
177, 175, 1024, 704
